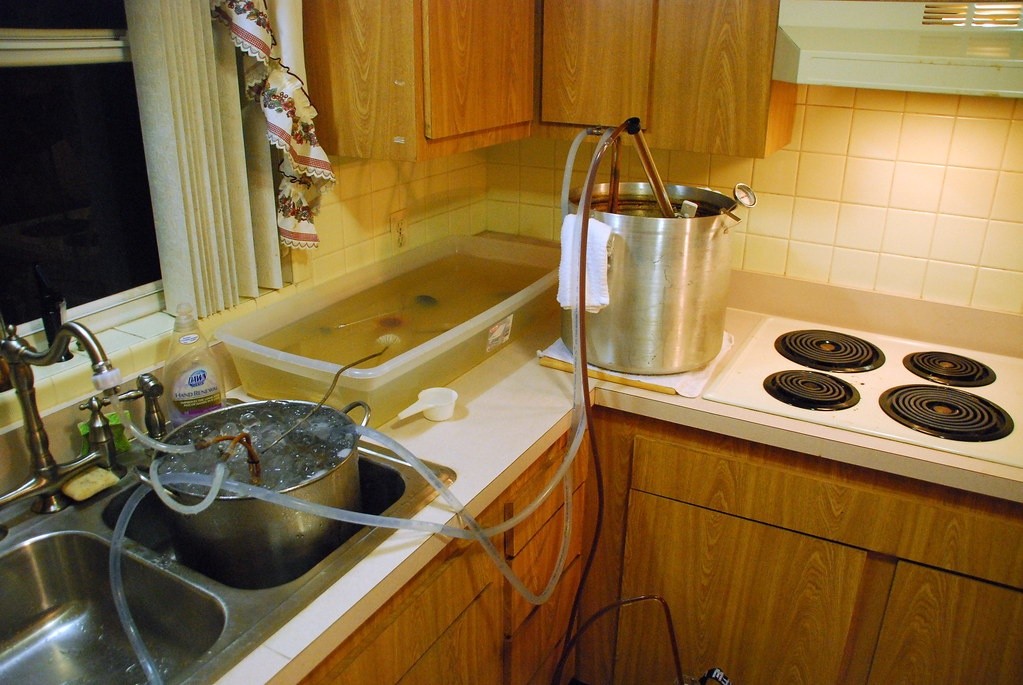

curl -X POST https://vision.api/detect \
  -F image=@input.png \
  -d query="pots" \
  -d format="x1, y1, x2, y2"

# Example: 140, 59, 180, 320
563, 182, 758, 378
133, 400, 371, 589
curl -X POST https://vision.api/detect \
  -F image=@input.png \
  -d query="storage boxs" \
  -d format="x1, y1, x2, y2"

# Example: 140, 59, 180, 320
213, 231, 560, 430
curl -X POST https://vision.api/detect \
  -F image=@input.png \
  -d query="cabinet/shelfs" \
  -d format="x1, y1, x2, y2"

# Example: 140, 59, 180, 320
610, 418, 1023, 685
530, 0, 798, 160
302, 0, 534, 164
302, 424, 590, 685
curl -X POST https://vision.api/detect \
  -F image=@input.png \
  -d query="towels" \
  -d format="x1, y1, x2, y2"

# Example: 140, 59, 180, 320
556, 214, 613, 315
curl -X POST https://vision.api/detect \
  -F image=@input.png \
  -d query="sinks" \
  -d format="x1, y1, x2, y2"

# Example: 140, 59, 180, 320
86, 398, 456, 598
0, 511, 248, 685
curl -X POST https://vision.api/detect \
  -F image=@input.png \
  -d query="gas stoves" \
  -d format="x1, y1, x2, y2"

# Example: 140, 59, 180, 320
763, 329, 1014, 442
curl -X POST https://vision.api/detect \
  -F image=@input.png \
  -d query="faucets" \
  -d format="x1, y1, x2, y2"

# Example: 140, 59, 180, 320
0, 321, 123, 541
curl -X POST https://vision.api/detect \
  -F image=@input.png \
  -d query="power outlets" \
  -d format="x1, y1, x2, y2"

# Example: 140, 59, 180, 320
390, 208, 410, 256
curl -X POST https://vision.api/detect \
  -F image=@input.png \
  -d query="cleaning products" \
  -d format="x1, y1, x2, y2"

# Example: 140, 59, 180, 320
160, 302, 226, 431
